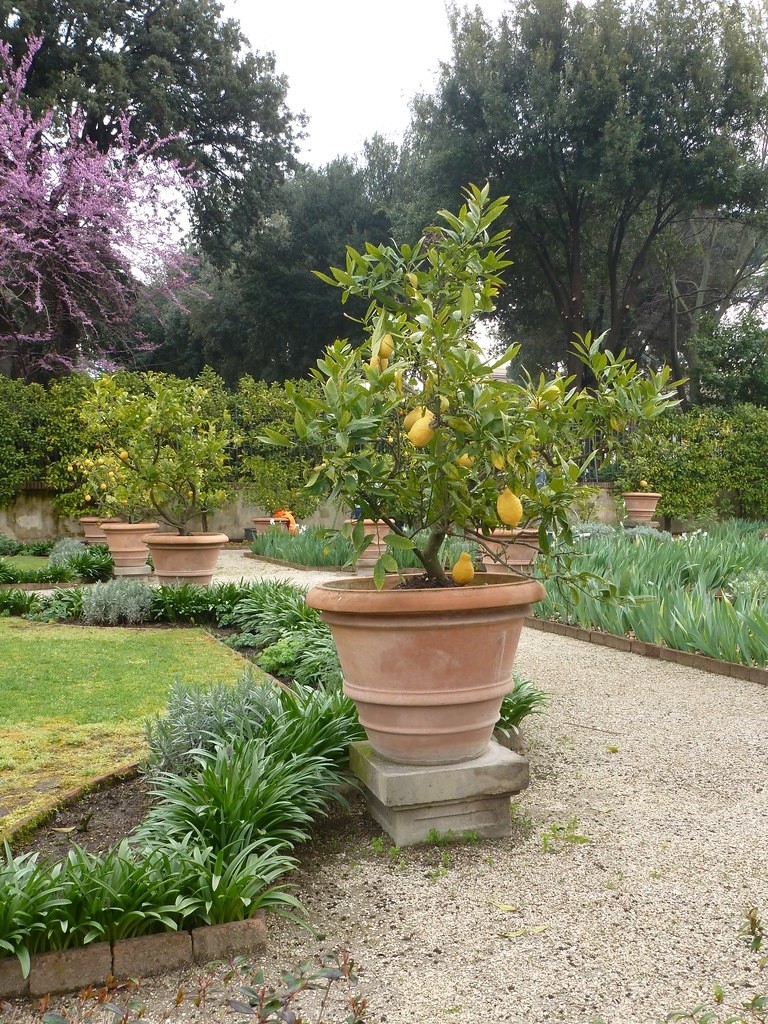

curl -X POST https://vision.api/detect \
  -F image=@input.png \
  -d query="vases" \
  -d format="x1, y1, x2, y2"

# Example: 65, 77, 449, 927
472, 527, 540, 576
618, 434, 662, 521
344, 518, 395, 567
256, 179, 689, 767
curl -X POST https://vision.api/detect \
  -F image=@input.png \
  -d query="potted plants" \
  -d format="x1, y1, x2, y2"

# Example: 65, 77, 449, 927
236, 455, 320, 538
68, 448, 127, 545
99, 451, 160, 567
80, 373, 229, 591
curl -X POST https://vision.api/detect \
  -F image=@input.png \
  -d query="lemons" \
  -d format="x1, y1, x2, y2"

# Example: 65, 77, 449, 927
639, 480, 647, 487
68, 451, 129, 501
369, 273, 559, 585
